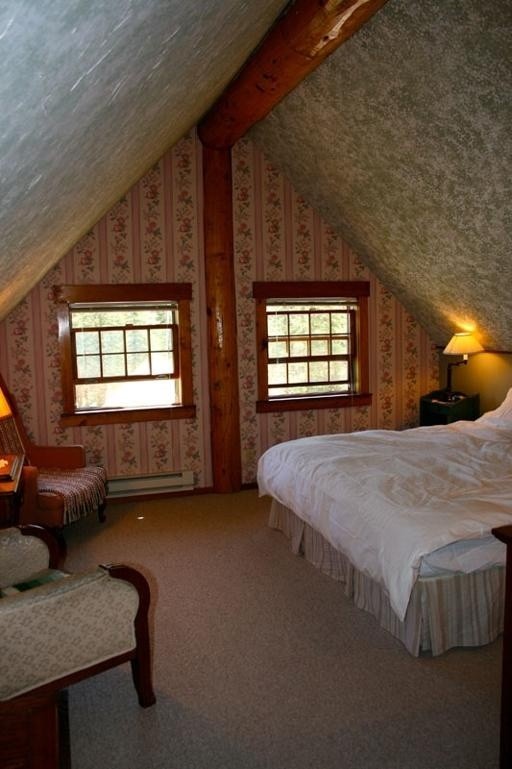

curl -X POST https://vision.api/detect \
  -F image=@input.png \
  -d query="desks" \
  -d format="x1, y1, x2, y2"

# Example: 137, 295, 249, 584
0, 453, 26, 528
490, 524, 512, 769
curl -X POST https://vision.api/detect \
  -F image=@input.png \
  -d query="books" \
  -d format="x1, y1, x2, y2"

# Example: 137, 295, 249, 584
0, 455, 18, 481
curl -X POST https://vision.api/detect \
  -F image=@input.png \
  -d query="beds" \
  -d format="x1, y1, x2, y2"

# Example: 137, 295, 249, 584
256, 373, 512, 660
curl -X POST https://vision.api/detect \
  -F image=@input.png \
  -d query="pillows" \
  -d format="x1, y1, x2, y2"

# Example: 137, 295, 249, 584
481, 387, 512, 430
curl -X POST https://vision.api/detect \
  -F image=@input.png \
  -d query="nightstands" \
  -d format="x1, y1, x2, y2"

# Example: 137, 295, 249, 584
419, 388, 480, 425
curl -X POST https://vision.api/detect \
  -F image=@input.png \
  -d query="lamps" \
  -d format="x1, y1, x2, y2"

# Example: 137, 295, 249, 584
0, 386, 13, 420
442, 332, 484, 401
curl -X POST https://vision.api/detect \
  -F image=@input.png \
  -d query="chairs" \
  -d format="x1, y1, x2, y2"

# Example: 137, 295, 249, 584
0, 524, 156, 769
0, 372, 108, 571
